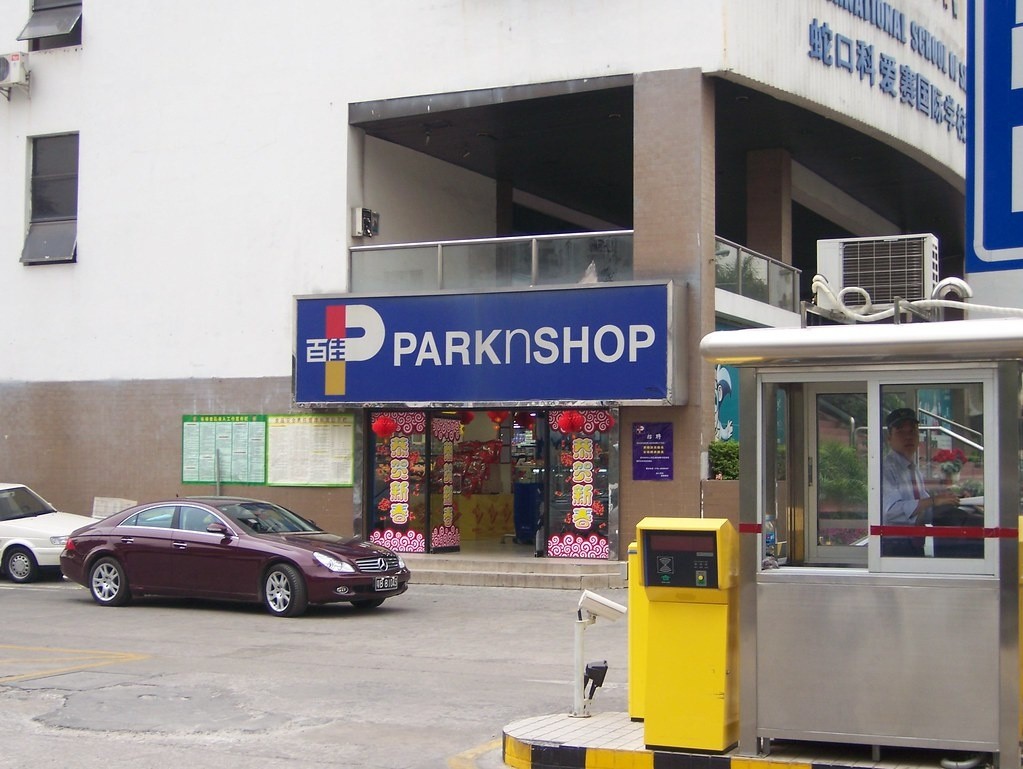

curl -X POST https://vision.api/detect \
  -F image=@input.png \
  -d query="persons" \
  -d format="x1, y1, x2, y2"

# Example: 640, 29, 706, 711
879, 407, 961, 558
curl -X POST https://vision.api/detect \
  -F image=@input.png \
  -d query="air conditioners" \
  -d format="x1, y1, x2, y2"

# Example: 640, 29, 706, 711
0, 51, 31, 88
817, 232, 938, 312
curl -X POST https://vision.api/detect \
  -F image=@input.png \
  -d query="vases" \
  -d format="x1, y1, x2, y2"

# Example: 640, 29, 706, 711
945, 470, 961, 482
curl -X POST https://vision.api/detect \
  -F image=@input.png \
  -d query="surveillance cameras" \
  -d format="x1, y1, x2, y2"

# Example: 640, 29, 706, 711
578, 589, 628, 623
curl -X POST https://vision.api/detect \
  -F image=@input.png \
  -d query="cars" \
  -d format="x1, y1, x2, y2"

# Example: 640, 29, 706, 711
59, 495, 410, 618
0, 482, 105, 584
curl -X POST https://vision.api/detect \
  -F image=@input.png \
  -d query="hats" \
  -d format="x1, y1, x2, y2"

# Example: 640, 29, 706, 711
886, 407, 920, 431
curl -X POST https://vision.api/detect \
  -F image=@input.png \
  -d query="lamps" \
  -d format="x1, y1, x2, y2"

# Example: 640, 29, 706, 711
583, 663, 608, 701
425, 131, 432, 147
461, 150, 472, 159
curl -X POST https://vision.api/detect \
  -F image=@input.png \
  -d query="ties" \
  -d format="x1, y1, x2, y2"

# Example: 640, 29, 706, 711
908, 463, 920, 500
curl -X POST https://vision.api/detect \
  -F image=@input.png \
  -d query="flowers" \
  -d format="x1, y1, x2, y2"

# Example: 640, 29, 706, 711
934, 447, 968, 475
